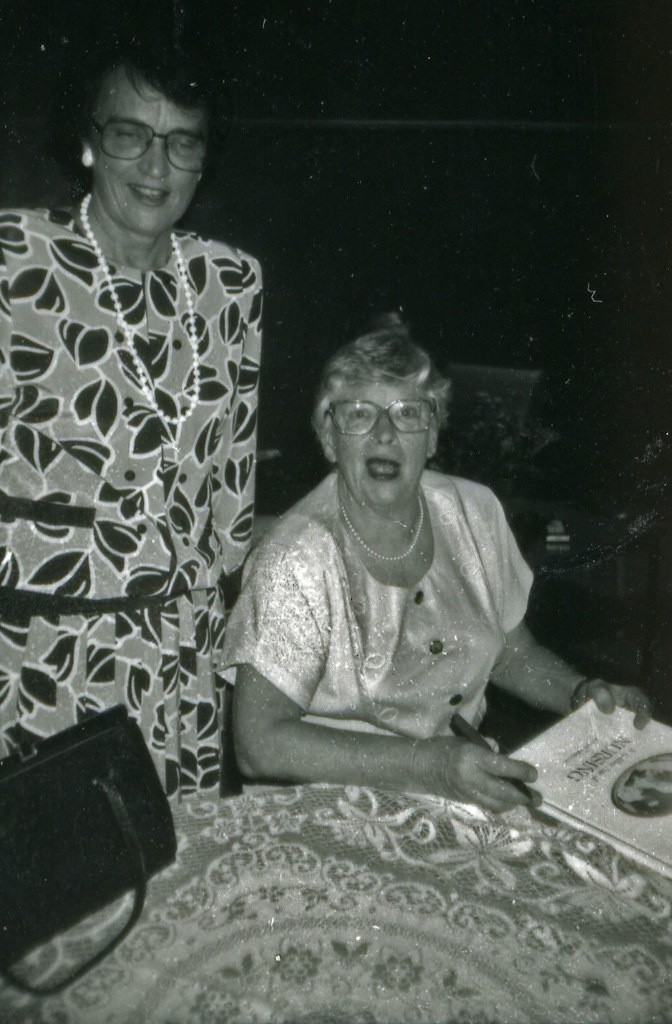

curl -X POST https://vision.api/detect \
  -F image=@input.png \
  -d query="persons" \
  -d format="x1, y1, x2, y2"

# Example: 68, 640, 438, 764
212, 310, 651, 815
1, 21, 265, 804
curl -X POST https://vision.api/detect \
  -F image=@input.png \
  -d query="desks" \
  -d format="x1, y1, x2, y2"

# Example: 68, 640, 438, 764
0, 785, 672, 1024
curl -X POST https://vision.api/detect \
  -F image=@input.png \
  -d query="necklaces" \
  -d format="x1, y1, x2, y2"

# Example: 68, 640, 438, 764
79, 189, 201, 424
338, 492, 425, 562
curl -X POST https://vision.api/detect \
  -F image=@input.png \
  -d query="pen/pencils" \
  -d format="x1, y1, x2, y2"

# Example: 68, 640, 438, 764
451, 712, 535, 799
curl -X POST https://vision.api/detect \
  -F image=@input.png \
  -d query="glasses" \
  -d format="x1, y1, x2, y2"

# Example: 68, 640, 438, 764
325, 398, 437, 436
83, 111, 215, 173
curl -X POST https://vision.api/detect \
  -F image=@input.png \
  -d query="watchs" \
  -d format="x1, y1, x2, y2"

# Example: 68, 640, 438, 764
568, 676, 597, 713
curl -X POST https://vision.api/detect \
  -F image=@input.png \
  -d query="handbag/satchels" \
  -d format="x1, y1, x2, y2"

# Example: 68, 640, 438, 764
1, 704, 177, 995
